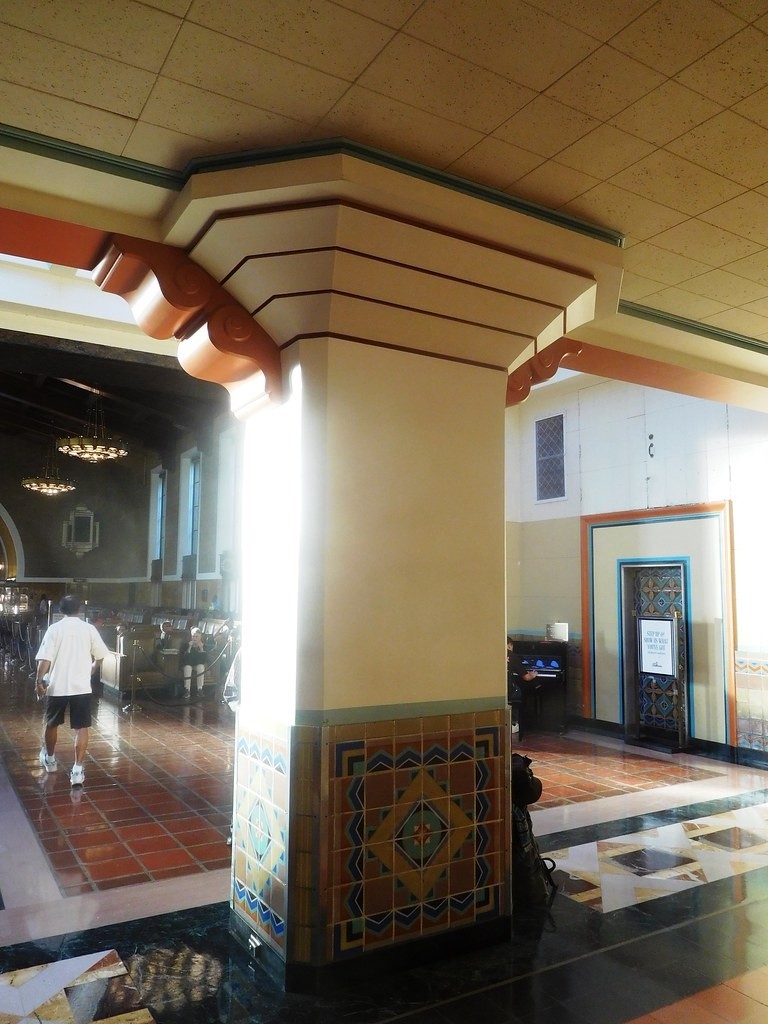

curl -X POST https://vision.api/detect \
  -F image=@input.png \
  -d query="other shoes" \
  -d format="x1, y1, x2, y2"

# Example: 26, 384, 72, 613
183, 693, 191, 698
196, 692, 205, 697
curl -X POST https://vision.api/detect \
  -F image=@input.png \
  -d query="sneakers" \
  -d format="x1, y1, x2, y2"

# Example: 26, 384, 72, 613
39, 749, 57, 773
70, 769, 84, 787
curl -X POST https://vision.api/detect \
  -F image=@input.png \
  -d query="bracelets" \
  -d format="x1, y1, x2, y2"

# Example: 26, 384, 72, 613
36, 680, 43, 684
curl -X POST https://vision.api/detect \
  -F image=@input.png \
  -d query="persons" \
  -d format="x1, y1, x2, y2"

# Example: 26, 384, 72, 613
39, 594, 59, 612
213, 618, 242, 655
160, 622, 189, 653
223, 648, 241, 844
35, 595, 109, 786
179, 628, 206, 699
116, 623, 126, 631
507, 637, 537, 733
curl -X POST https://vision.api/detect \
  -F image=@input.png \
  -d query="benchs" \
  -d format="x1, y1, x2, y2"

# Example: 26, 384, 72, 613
99, 622, 222, 702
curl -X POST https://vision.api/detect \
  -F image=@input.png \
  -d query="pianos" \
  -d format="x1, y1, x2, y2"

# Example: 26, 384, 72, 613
505, 635, 571, 732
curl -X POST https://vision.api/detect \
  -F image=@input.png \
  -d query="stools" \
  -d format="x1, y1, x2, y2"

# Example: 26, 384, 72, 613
508, 699, 525, 741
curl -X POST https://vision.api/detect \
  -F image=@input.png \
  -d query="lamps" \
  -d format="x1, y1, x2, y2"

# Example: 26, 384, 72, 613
56, 395, 130, 464
20, 419, 80, 496
